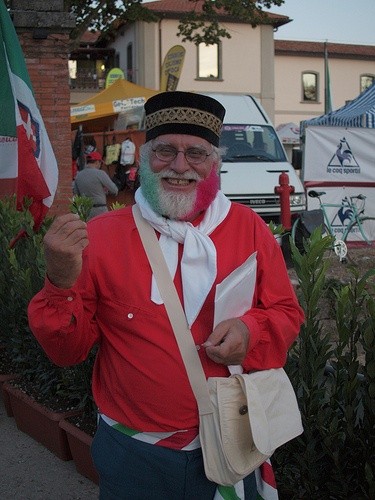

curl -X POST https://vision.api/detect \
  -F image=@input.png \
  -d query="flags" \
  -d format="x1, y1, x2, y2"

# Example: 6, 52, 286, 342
0, 0, 59, 250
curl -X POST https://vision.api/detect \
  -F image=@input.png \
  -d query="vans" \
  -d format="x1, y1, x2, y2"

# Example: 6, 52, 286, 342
186, 92, 308, 221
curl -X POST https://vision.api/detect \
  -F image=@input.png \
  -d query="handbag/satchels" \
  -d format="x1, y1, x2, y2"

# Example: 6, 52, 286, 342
198, 367, 304, 486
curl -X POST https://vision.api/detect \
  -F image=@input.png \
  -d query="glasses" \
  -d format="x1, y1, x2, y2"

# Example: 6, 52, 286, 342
150, 143, 215, 165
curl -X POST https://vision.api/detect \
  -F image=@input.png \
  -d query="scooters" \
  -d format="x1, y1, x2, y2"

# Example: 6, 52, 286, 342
111, 160, 142, 192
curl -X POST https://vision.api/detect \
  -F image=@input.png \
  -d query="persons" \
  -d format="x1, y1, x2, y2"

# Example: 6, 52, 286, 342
26, 91, 304, 500
72, 151, 118, 223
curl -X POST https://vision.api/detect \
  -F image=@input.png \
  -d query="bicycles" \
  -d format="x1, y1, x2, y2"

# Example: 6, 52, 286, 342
291, 190, 375, 268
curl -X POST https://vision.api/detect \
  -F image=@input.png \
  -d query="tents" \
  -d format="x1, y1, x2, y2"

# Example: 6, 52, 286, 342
275, 87, 375, 249
69, 76, 162, 169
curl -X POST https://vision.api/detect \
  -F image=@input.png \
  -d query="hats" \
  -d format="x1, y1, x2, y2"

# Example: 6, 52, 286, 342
144, 90, 226, 148
86, 151, 103, 161
